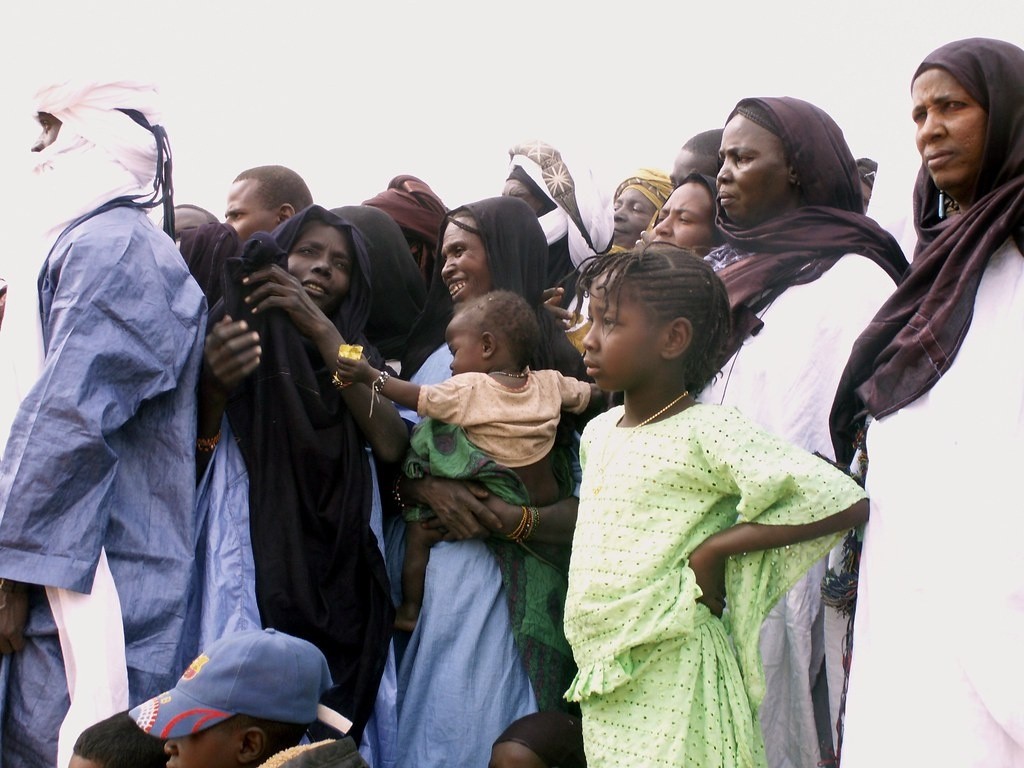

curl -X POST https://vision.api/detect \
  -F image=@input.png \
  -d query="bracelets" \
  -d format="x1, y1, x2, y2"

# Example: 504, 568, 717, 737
197, 431, 222, 452
392, 475, 405, 510
501, 507, 539, 543
331, 372, 354, 390
369, 372, 389, 418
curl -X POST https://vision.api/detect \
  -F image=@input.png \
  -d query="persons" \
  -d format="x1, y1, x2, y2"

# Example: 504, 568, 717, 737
225, 164, 312, 248
0, 36, 453, 768
487, 709, 587, 768
497, 136, 616, 360
564, 38, 1024, 768
355, 194, 591, 768
333, 288, 604, 638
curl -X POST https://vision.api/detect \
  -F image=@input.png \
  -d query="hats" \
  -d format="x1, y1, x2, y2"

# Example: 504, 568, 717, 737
128, 626, 333, 738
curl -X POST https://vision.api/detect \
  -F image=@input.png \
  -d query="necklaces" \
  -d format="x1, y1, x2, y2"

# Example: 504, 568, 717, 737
591, 390, 690, 496
488, 371, 525, 379
